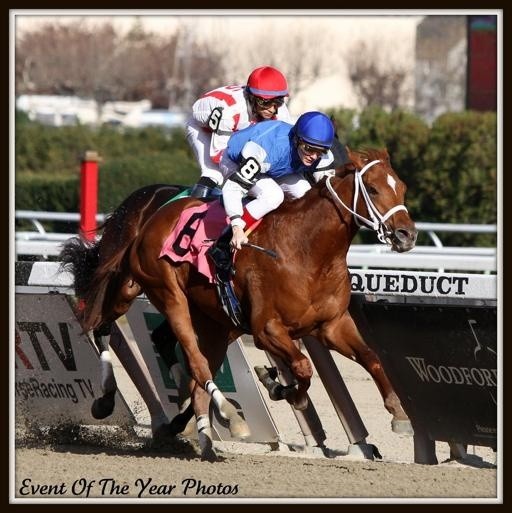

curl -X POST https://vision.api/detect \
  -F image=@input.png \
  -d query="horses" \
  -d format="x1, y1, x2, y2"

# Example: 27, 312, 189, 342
56, 143, 419, 464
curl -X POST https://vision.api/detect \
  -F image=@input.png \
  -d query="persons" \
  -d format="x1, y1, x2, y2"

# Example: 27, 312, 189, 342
208, 111, 336, 271
184, 66, 292, 199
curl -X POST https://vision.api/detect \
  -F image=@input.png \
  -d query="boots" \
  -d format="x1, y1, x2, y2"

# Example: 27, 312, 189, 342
190, 184, 213, 197
207, 223, 247, 277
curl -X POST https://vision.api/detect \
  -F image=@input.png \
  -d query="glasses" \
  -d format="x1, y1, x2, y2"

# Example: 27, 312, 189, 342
257, 98, 284, 108
300, 140, 324, 158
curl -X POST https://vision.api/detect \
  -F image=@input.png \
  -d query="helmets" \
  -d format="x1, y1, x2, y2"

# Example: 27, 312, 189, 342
293, 111, 334, 154
247, 66, 289, 102
